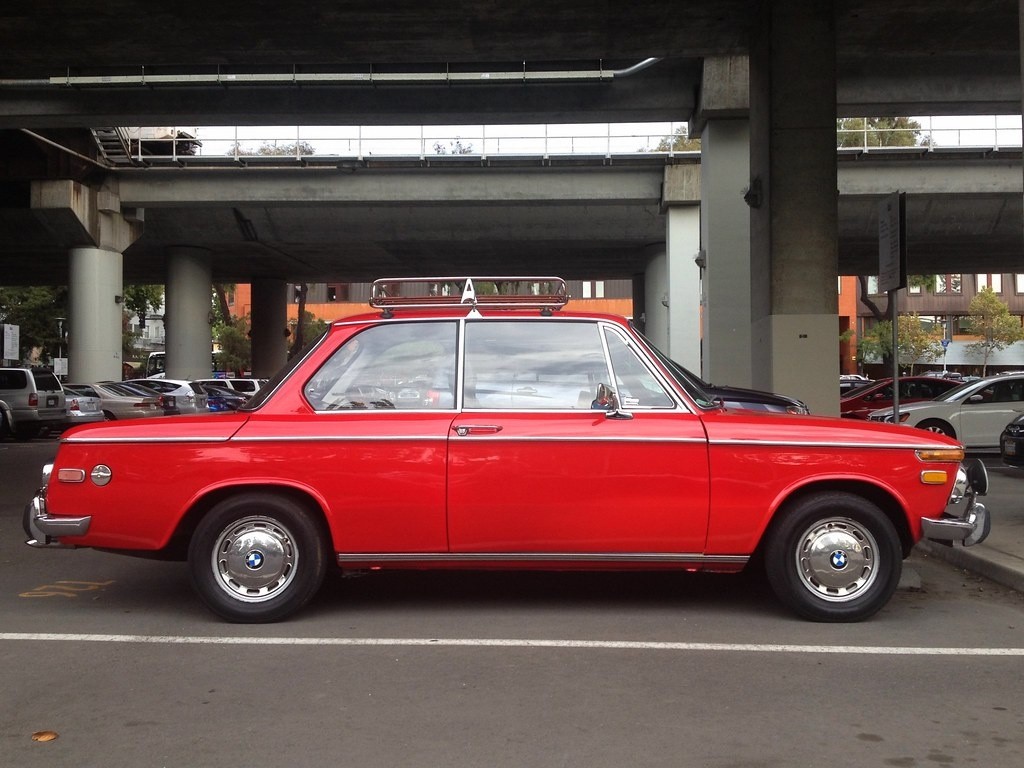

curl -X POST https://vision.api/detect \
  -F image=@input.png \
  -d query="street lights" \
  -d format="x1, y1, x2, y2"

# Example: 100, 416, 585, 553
940, 317, 949, 375
54, 317, 67, 383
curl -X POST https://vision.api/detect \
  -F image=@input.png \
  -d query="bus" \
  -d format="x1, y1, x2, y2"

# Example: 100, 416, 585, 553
145, 351, 252, 379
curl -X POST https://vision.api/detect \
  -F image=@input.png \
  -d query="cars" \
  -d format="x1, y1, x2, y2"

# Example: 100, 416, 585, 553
35, 386, 105, 439
863, 372, 1024, 449
21, 273, 995, 628
61, 380, 165, 421
840, 376, 994, 419
96, 377, 270, 417
840, 370, 1024, 393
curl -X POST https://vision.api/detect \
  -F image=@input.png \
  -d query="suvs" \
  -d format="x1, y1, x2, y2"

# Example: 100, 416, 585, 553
0, 365, 69, 443
